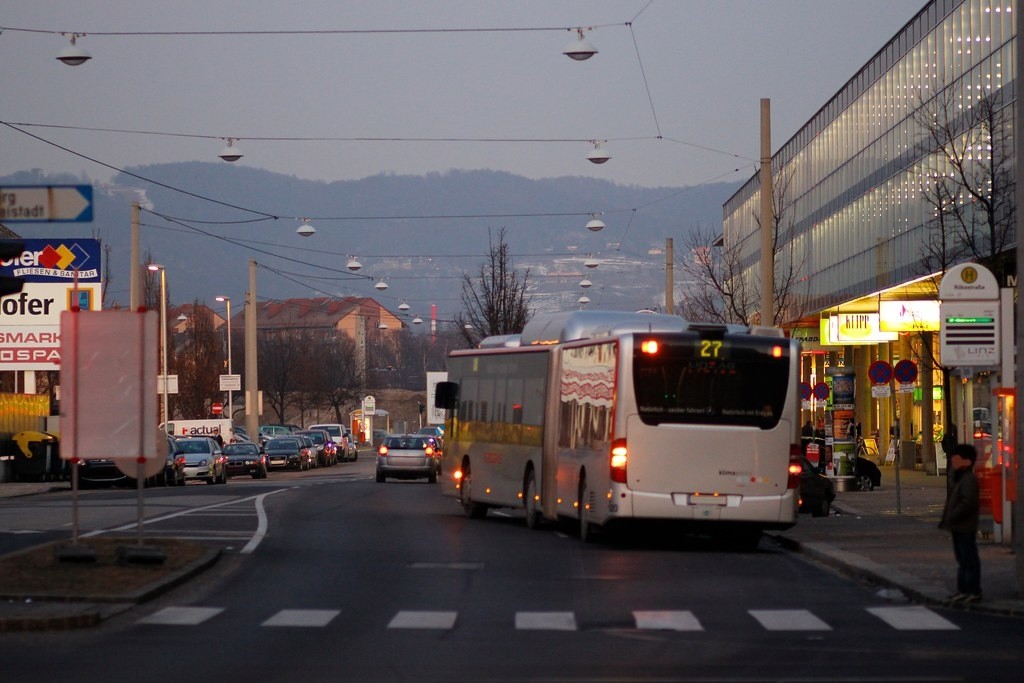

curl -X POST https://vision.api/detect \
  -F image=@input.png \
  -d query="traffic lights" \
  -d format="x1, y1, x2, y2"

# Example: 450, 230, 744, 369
419, 403, 425, 413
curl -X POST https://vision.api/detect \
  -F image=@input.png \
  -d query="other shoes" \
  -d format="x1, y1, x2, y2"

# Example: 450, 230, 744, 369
950, 592, 968, 602
962, 594, 983, 605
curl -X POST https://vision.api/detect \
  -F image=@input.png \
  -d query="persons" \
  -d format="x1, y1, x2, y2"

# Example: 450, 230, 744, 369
213, 429, 223, 445
849, 425, 855, 438
814, 428, 826, 439
936, 444, 982, 602
856, 422, 861, 438
802, 420, 813, 437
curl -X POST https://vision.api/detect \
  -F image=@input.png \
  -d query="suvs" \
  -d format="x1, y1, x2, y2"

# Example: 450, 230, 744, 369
293, 430, 337, 467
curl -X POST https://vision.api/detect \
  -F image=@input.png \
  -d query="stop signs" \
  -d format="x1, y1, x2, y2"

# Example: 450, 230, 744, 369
212, 403, 223, 414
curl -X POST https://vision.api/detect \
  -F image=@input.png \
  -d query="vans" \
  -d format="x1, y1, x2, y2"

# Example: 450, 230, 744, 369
309, 424, 349, 463
158, 418, 233, 446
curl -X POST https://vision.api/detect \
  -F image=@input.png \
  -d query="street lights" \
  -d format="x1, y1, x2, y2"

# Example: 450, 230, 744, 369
216, 295, 232, 419
148, 264, 169, 435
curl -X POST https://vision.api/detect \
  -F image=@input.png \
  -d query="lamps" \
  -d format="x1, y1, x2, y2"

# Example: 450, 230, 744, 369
49, 32, 612, 330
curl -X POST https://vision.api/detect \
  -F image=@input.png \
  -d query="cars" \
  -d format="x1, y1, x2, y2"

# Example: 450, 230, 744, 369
802, 437, 881, 492
221, 443, 267, 480
264, 435, 317, 471
376, 427, 444, 484
348, 435, 358, 461
175, 437, 225, 485
76, 435, 185, 487
234, 424, 302, 444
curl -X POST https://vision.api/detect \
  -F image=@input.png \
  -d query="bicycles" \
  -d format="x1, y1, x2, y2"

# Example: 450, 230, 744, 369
855, 437, 876, 456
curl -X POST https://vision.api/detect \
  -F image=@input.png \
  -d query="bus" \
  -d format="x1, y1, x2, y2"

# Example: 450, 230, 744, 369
436, 312, 801, 541
